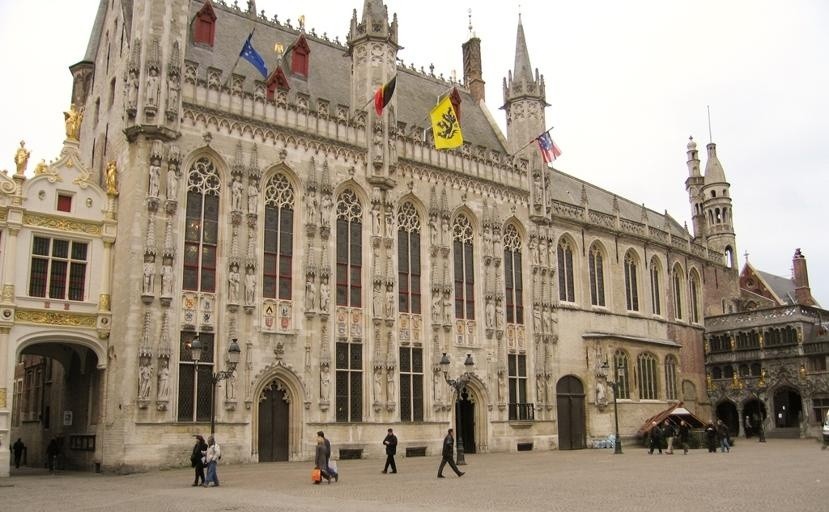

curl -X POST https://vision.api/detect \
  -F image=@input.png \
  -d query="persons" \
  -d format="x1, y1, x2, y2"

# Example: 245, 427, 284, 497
317, 431, 338, 482
200, 436, 221, 487
313, 437, 331, 484
190, 435, 207, 486
381, 428, 399, 474
14, 141, 30, 174
648, 417, 689, 456
13, 437, 24, 468
745, 415, 752, 439
437, 428, 466, 477
715, 419, 731, 453
46, 439, 58, 472
705, 420, 718, 453
105, 159, 120, 197
64, 102, 83, 139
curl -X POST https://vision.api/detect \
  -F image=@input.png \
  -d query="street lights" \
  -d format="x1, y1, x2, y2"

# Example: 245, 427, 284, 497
191, 334, 241, 442
745, 379, 771, 443
439, 352, 475, 466
601, 363, 624, 455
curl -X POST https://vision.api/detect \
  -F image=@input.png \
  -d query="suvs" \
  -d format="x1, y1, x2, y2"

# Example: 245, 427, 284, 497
822, 410, 829, 444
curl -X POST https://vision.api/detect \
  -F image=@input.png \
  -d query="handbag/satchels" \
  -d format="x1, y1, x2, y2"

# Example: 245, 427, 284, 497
312, 467, 321, 481
328, 459, 336, 473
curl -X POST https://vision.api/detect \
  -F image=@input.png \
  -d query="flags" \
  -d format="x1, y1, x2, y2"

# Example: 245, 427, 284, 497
239, 41, 268, 79
535, 131, 561, 165
430, 97, 463, 149
374, 78, 397, 117
